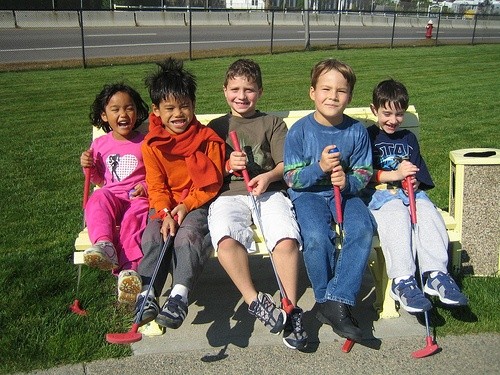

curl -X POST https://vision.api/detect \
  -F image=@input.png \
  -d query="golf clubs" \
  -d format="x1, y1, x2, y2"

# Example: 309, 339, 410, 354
407, 173, 441, 358
105, 181, 191, 344
329, 147, 357, 353
230, 131, 294, 313
69, 150, 95, 315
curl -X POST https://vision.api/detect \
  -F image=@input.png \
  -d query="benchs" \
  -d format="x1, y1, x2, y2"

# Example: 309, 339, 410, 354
72, 104, 462, 336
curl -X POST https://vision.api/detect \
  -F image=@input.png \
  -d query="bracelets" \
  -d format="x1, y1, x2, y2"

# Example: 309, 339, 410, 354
226, 158, 242, 177
151, 207, 171, 221
375, 169, 384, 184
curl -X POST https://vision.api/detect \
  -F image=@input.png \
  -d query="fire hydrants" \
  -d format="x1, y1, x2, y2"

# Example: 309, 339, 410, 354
424, 19, 434, 39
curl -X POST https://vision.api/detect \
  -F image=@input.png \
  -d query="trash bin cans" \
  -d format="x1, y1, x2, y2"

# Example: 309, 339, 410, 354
448, 148, 500, 277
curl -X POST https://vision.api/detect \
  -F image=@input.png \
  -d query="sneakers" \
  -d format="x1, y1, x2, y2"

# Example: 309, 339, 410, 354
423, 271, 466, 305
155, 294, 190, 329
312, 298, 364, 343
247, 290, 287, 336
282, 305, 309, 351
391, 275, 433, 313
132, 294, 159, 328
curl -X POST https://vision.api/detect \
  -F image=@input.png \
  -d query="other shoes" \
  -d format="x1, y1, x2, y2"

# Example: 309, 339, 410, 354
85, 239, 120, 272
117, 269, 142, 306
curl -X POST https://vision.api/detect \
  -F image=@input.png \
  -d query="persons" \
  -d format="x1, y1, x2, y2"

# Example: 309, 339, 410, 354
135, 55, 225, 330
206, 57, 309, 351
362, 77, 469, 313
284, 58, 372, 342
81, 83, 150, 311
